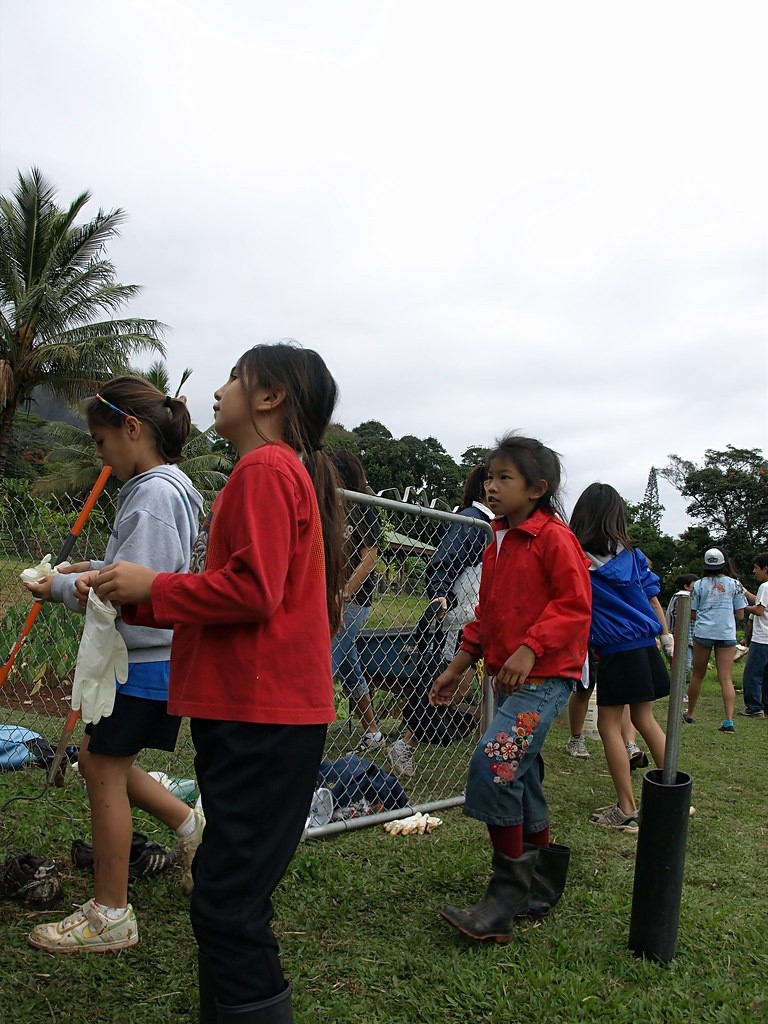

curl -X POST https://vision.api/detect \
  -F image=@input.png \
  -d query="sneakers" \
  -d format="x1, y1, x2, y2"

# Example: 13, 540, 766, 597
565, 734, 590, 758
385, 741, 416, 782
1, 853, 65, 912
181, 809, 208, 896
625, 741, 649, 771
127, 831, 175, 877
588, 804, 639, 834
346, 734, 387, 756
28, 899, 139, 952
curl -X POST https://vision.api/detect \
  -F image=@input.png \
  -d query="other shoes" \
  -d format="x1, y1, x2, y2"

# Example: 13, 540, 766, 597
683, 695, 689, 702
71, 839, 93, 868
681, 709, 694, 724
148, 771, 199, 804
733, 643, 749, 662
737, 707, 765, 719
718, 723, 734, 733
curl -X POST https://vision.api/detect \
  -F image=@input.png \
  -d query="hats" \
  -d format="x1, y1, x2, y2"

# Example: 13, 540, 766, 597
703, 545, 727, 570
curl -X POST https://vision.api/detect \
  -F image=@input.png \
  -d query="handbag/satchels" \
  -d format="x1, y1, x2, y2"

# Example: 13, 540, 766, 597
400, 602, 482, 747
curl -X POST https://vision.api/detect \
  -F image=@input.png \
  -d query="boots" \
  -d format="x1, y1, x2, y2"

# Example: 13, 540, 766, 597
516, 842, 571, 919
440, 843, 540, 944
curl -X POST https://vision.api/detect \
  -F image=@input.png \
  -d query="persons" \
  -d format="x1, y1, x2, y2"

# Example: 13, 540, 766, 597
428, 427, 593, 942
27, 376, 206, 952
326, 449, 768, 835
73, 340, 342, 1024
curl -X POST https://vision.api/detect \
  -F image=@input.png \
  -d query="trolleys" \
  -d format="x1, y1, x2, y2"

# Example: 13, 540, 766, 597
355, 626, 433, 696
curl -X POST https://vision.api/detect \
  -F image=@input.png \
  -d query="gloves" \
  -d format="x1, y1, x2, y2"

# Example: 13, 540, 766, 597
659, 634, 674, 669
385, 812, 443, 836
72, 586, 129, 725
20, 553, 71, 586
